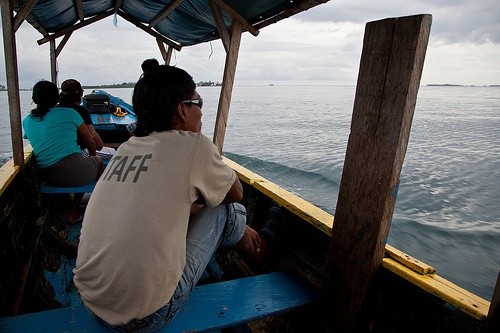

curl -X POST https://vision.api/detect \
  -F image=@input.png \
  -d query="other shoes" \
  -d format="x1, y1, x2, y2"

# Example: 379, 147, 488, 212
67, 211, 84, 224
46, 206, 60, 221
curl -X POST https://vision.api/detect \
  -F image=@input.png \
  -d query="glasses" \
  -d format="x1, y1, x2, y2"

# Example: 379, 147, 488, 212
181, 93, 203, 110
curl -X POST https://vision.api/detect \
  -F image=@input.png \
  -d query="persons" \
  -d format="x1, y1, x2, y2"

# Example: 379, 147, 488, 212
21, 78, 106, 224
141, 59, 158, 71
72, 65, 262, 333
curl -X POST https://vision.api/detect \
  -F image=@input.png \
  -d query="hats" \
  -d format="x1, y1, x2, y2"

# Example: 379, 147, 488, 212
61, 79, 84, 94
